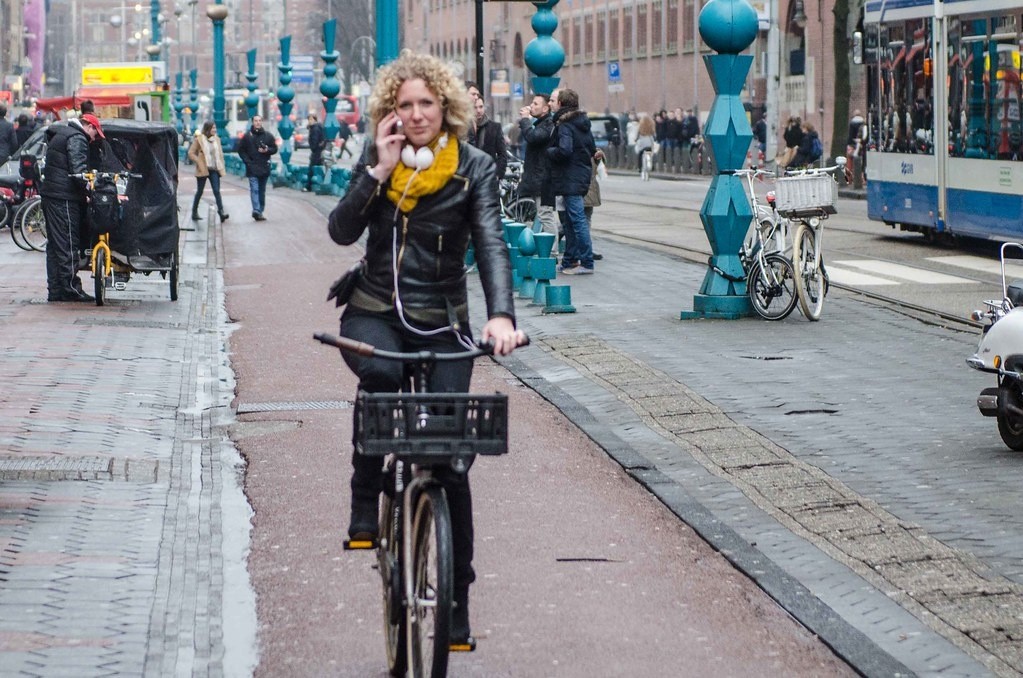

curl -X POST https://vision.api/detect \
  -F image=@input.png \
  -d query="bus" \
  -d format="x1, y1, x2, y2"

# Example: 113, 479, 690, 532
199, 90, 280, 145
322, 97, 361, 134
850, 3, 1023, 251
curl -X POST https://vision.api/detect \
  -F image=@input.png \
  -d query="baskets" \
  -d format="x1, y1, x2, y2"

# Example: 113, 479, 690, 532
771, 176, 839, 219
353, 389, 509, 461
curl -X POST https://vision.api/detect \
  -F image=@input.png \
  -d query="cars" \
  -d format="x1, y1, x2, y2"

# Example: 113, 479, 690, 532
0, 123, 48, 228
589, 116, 623, 154
293, 118, 311, 150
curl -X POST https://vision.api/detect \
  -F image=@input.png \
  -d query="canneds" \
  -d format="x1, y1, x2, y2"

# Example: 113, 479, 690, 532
525, 106, 531, 110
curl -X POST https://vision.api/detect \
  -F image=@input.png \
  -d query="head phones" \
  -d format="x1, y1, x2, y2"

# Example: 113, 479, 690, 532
402, 125, 450, 171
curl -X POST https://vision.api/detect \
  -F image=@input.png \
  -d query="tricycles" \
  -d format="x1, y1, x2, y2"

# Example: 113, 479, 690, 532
68, 120, 181, 306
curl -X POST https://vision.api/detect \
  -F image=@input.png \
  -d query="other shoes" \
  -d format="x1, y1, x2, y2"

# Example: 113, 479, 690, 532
562, 265, 594, 276
47, 293, 94, 303
447, 581, 469, 645
591, 251, 603, 261
253, 212, 266, 220
347, 491, 379, 540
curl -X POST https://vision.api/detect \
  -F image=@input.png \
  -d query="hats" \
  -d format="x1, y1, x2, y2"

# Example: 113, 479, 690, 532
82, 114, 105, 140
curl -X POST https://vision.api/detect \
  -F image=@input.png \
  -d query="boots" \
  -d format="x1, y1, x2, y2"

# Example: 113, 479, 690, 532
192, 206, 203, 222
218, 208, 229, 222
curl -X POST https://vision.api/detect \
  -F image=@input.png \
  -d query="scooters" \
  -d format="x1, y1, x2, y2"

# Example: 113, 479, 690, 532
966, 240, 1022, 451
321, 144, 334, 170
689, 137, 712, 171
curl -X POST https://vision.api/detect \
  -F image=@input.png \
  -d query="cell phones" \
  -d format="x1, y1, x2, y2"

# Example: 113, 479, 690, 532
392, 109, 404, 135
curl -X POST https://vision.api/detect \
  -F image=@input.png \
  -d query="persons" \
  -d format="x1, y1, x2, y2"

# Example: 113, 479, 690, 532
188, 121, 230, 222
518, 88, 607, 275
326, 55, 517, 643
753, 111, 767, 153
337, 118, 353, 159
0, 101, 19, 167
237, 116, 278, 220
653, 108, 700, 148
301, 114, 325, 192
464, 80, 508, 185
79, 101, 100, 119
40, 114, 106, 302
849, 109, 867, 181
784, 116, 818, 167
16, 115, 34, 146
356, 116, 366, 134
620, 106, 656, 173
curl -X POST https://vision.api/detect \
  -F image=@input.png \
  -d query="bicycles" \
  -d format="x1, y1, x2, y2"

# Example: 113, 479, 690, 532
718, 166, 844, 322
499, 153, 536, 227
310, 329, 530, 678
7, 142, 49, 253
640, 149, 653, 180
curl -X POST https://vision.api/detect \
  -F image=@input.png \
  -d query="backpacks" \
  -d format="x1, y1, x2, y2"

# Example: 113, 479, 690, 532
88, 181, 119, 233
807, 134, 823, 156
